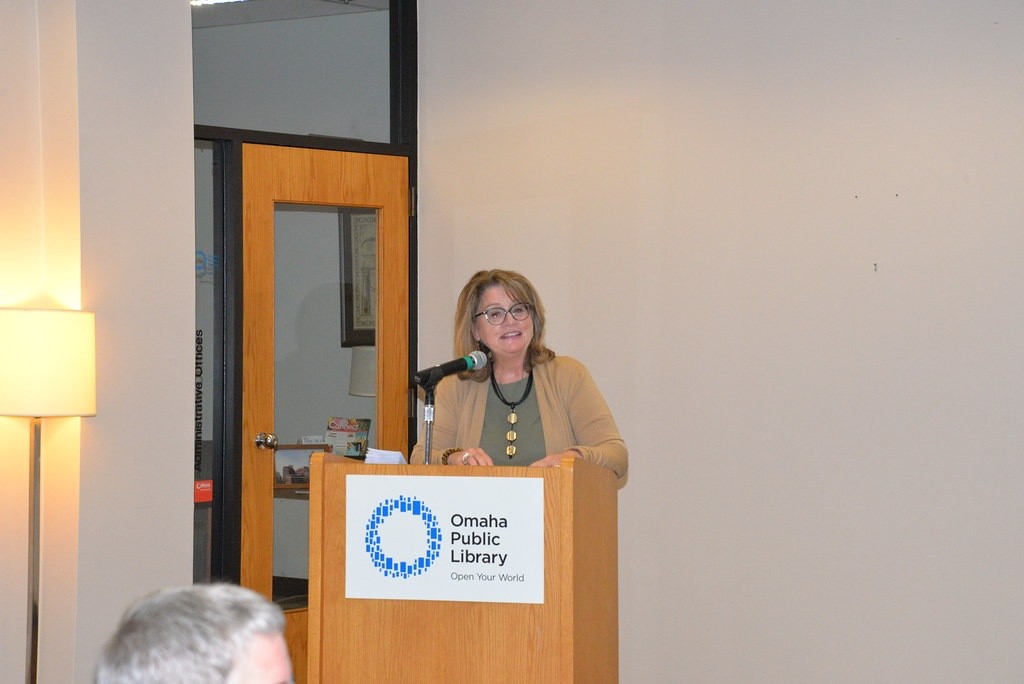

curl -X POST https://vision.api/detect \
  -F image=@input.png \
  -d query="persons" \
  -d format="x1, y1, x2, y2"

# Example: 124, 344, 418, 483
410, 269, 628, 479
95, 582, 294, 684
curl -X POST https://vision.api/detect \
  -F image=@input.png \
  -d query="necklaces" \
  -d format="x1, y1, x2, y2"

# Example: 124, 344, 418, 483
490, 363, 533, 460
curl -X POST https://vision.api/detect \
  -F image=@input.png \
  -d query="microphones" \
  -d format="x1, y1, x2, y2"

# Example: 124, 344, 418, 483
412, 351, 487, 385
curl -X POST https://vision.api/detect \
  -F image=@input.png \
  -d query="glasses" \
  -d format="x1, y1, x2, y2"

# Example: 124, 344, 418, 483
473, 301, 530, 325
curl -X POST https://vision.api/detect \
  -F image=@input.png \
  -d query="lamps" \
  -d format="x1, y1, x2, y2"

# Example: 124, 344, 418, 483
349, 346, 377, 398
1, 308, 98, 684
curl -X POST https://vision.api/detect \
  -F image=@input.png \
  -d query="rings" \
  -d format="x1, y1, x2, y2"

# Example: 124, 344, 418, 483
463, 460, 467, 465
464, 454, 469, 460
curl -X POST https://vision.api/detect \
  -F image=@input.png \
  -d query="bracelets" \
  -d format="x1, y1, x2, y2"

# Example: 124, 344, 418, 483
441, 448, 463, 465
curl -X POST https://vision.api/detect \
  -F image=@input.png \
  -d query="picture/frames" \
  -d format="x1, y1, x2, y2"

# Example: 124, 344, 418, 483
338, 211, 378, 347
